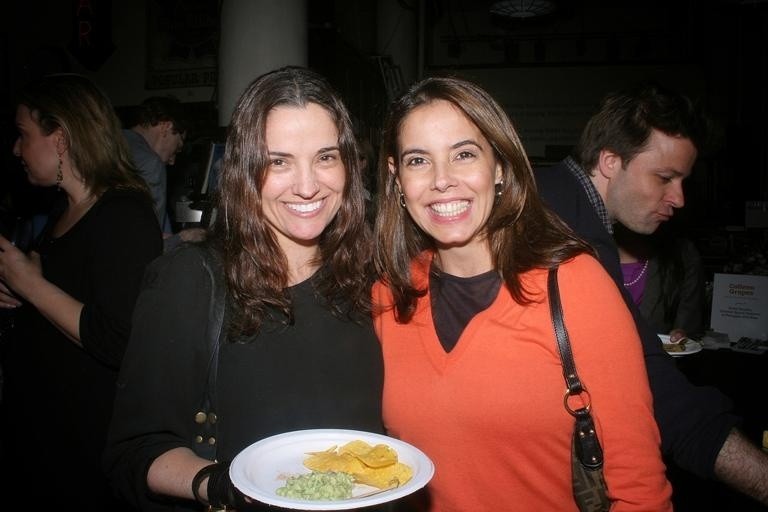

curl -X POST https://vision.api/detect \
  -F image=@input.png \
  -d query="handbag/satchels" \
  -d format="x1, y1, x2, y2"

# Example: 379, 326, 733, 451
571, 411, 610, 511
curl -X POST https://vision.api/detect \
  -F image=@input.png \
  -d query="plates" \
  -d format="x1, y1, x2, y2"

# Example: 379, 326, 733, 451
654, 333, 703, 357
229, 428, 436, 512
